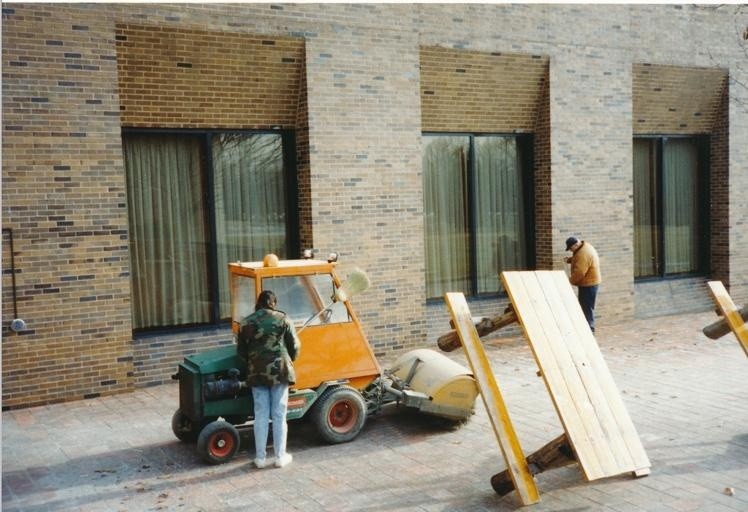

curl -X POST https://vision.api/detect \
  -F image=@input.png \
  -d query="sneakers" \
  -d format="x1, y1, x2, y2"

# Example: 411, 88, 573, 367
251, 451, 267, 469
273, 452, 293, 467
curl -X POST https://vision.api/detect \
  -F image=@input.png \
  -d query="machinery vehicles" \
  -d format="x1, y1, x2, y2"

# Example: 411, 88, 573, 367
170, 247, 484, 467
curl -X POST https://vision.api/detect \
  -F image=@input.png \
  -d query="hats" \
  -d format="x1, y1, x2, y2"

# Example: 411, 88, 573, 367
564, 237, 579, 251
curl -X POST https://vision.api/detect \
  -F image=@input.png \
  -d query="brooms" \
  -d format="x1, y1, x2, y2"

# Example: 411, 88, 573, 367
295, 267, 371, 339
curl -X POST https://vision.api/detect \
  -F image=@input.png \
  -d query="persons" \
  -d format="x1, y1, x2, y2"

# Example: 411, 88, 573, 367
563, 237, 601, 338
237, 292, 301, 468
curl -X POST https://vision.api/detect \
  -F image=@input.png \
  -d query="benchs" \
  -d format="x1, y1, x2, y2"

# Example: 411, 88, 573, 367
443, 289, 542, 509
706, 276, 746, 354
631, 469, 653, 479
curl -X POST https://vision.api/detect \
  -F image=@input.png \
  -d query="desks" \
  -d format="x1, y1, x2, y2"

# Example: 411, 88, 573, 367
437, 266, 654, 496
